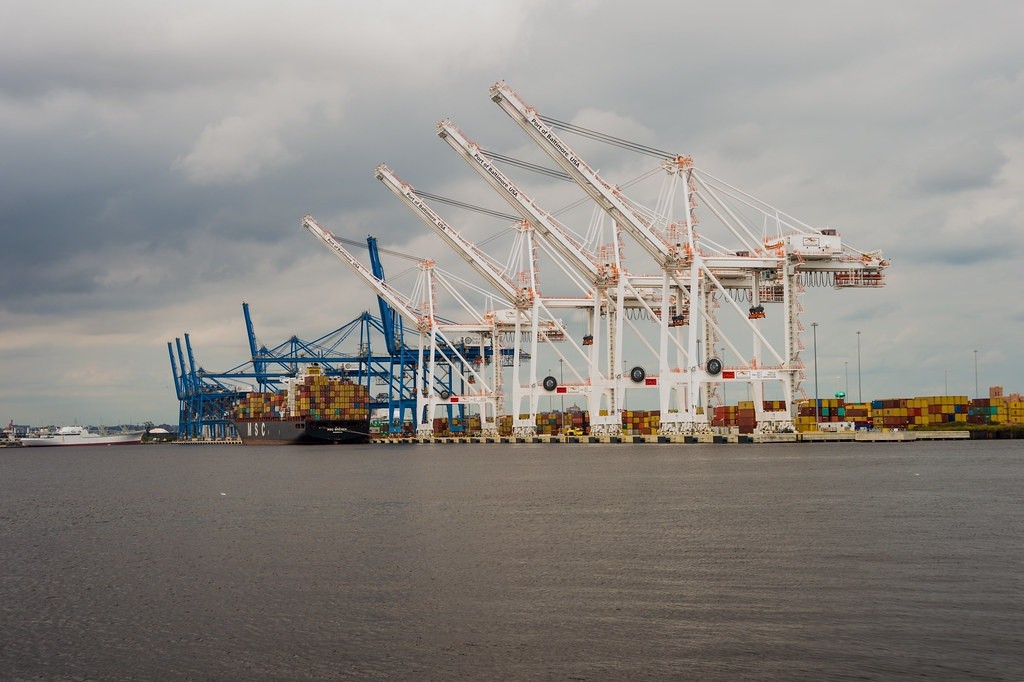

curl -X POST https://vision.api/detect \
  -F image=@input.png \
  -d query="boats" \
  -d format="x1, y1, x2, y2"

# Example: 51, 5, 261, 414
20, 425, 148, 446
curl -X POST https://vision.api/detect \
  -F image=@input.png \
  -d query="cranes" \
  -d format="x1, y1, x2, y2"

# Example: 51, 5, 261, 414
299, 79, 888, 429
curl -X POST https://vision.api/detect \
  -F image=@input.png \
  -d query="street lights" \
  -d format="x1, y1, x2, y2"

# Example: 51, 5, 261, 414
810, 322, 819, 430
856, 330, 863, 405
845, 361, 849, 400
975, 350, 979, 399
